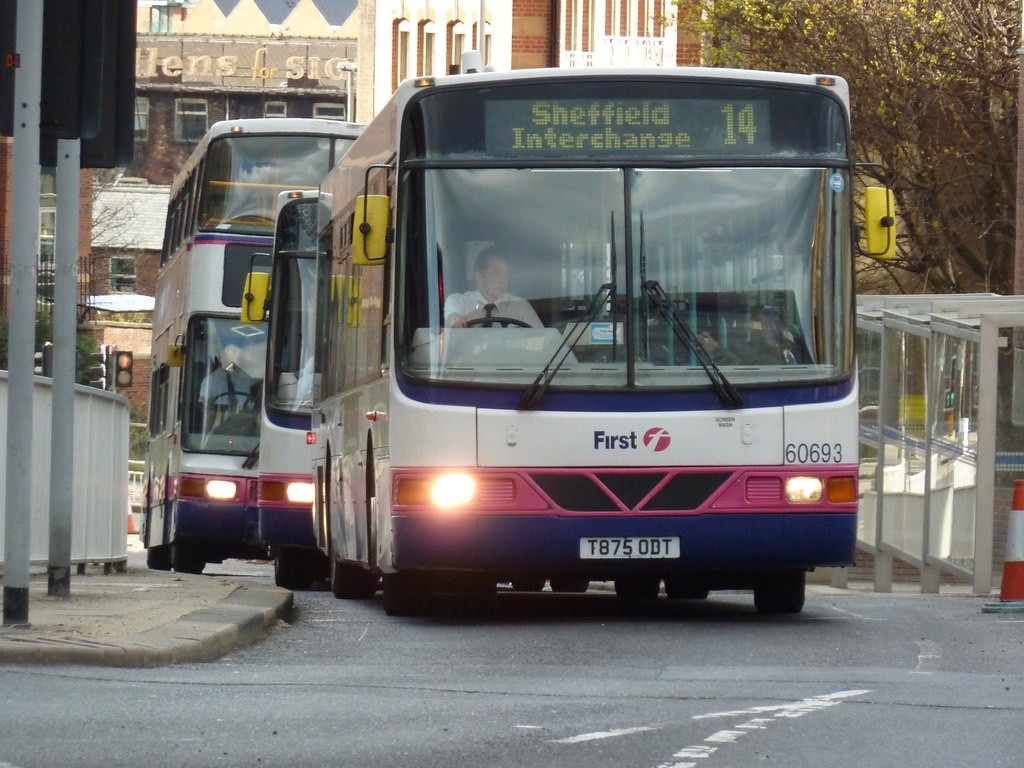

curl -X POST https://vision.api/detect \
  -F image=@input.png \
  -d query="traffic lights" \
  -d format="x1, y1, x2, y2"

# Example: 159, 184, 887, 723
88, 344, 111, 391
112, 350, 133, 388
34, 342, 52, 378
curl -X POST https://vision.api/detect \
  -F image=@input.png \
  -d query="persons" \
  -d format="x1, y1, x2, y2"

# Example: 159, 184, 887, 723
442, 245, 544, 328
694, 303, 802, 366
196, 342, 253, 436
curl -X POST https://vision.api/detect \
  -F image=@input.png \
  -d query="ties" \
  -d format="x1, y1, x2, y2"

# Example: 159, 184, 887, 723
481, 304, 494, 328
223, 371, 238, 414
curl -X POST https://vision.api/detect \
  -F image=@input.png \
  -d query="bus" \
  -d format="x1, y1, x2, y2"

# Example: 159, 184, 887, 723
309, 62, 899, 622
238, 190, 323, 588
138, 117, 374, 579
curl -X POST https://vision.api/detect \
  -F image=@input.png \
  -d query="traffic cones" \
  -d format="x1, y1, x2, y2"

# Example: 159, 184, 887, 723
981, 478, 1024, 613
